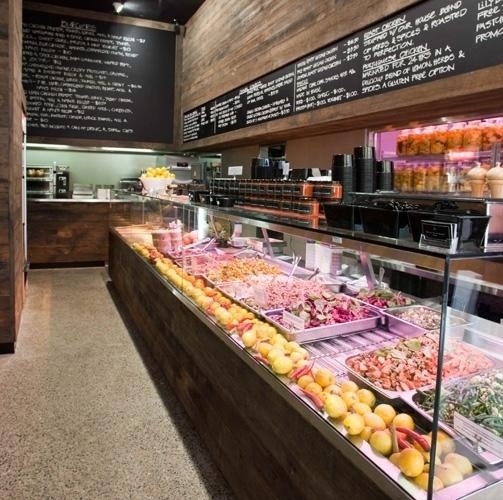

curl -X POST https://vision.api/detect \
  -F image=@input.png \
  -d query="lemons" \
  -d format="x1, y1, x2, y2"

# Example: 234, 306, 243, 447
132, 243, 473, 492
146, 166, 176, 178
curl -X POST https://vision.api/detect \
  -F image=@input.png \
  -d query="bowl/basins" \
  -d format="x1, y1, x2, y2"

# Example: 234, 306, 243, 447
198, 193, 236, 207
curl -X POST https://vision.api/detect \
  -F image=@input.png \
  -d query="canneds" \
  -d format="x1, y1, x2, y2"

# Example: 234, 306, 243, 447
189, 179, 342, 218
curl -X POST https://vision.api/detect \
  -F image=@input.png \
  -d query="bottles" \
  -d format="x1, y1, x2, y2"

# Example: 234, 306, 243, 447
464, 117, 502, 151
393, 162, 450, 194
420, 127, 433, 155
407, 129, 420, 156
447, 123, 463, 150
396, 129, 408, 156
432, 127, 446, 154
